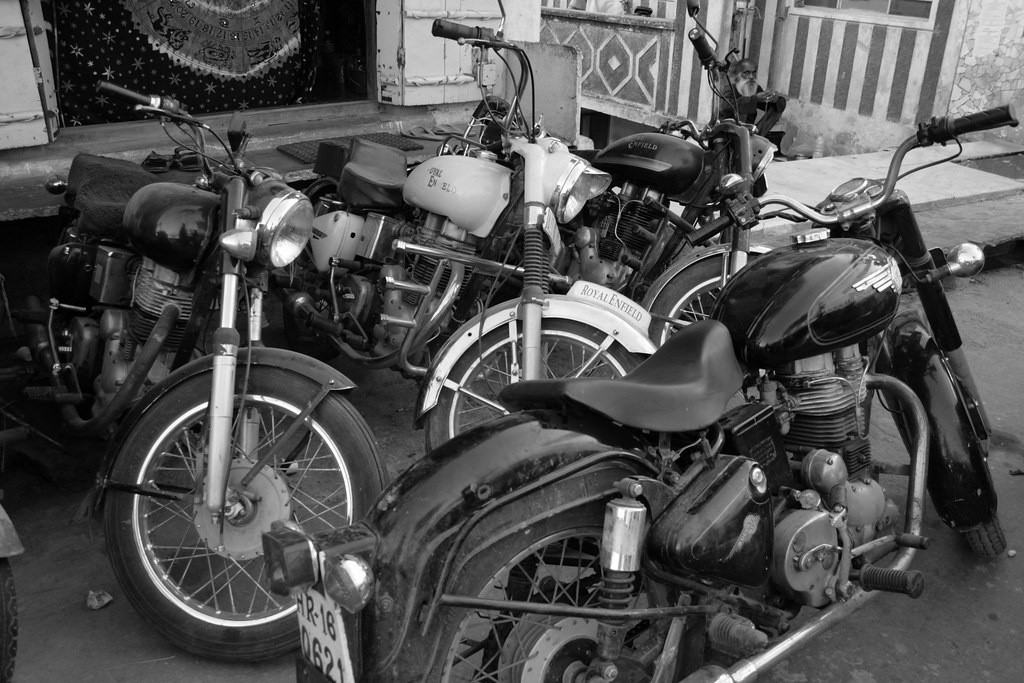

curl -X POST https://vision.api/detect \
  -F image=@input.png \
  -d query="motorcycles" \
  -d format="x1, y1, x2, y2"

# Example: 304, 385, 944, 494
568, 0, 786, 350
260, 104, 1020, 683
0, 80, 390, 683
245, 0, 658, 458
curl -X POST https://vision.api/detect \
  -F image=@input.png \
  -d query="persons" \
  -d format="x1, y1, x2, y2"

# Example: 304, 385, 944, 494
569, 0, 633, 149
